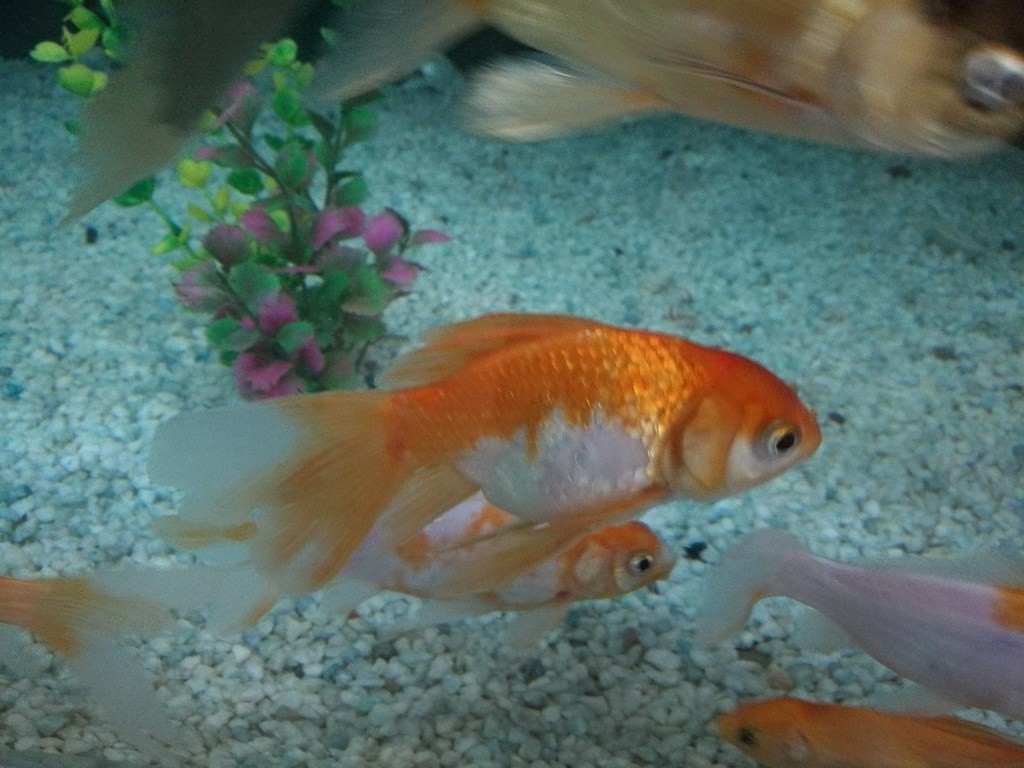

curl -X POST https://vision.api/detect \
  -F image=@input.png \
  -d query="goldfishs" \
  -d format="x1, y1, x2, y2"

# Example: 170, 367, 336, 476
53, 2, 1024, 226
0, 312, 821, 768
695, 527, 1024, 768
